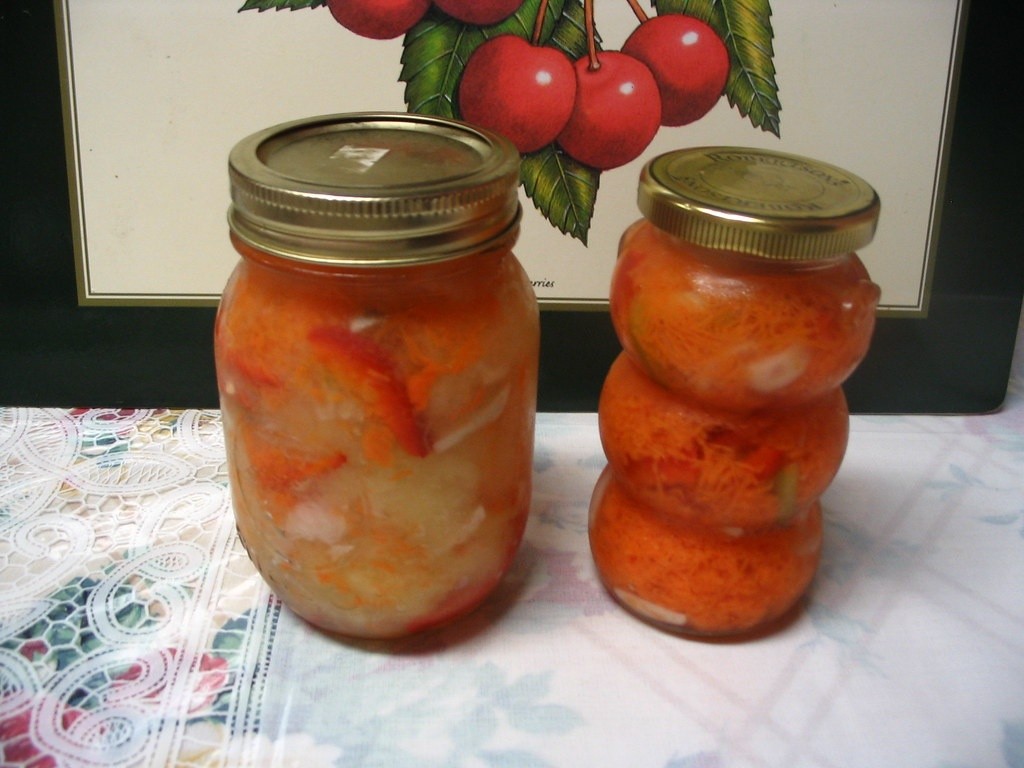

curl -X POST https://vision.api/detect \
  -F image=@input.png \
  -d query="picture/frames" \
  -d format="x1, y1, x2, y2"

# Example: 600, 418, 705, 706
0, 0, 1024, 417
